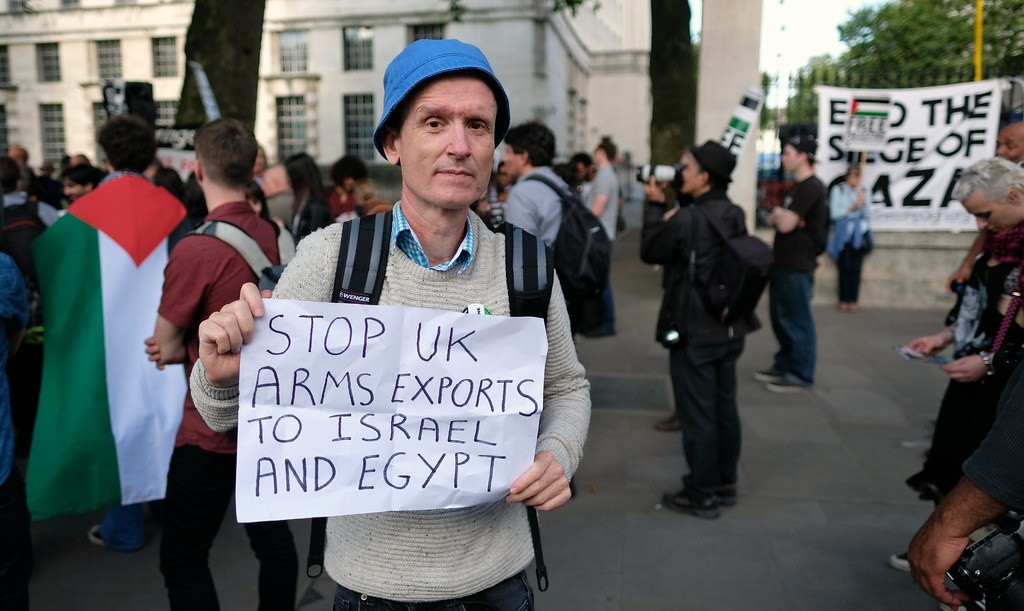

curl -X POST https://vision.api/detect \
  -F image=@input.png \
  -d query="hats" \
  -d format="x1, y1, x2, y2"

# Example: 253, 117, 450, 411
373, 38, 510, 164
787, 134, 818, 154
691, 140, 737, 184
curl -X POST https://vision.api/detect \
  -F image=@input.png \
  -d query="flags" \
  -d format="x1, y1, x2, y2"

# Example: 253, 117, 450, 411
26, 176, 190, 522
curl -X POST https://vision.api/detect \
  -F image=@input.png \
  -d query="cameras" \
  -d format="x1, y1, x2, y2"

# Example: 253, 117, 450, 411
635, 163, 687, 189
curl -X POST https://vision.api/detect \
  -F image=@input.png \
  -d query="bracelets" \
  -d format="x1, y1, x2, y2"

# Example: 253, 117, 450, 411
979, 350, 995, 375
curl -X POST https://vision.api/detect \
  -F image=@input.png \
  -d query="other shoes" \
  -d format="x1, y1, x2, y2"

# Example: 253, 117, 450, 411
839, 306, 857, 314
655, 413, 682, 431
661, 494, 722, 519
88, 525, 148, 551
767, 382, 807, 394
754, 370, 790, 383
705, 485, 737, 505
887, 554, 915, 572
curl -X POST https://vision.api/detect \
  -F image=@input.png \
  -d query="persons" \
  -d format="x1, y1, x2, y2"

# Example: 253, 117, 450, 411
752, 133, 829, 394
829, 165, 870, 312
189, 39, 592, 611
475, 121, 763, 517
889, 119, 1024, 611
0, 114, 394, 611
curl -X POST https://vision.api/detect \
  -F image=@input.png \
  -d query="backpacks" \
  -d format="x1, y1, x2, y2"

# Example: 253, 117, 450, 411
697, 206, 775, 327
188, 219, 297, 294
521, 174, 617, 337
